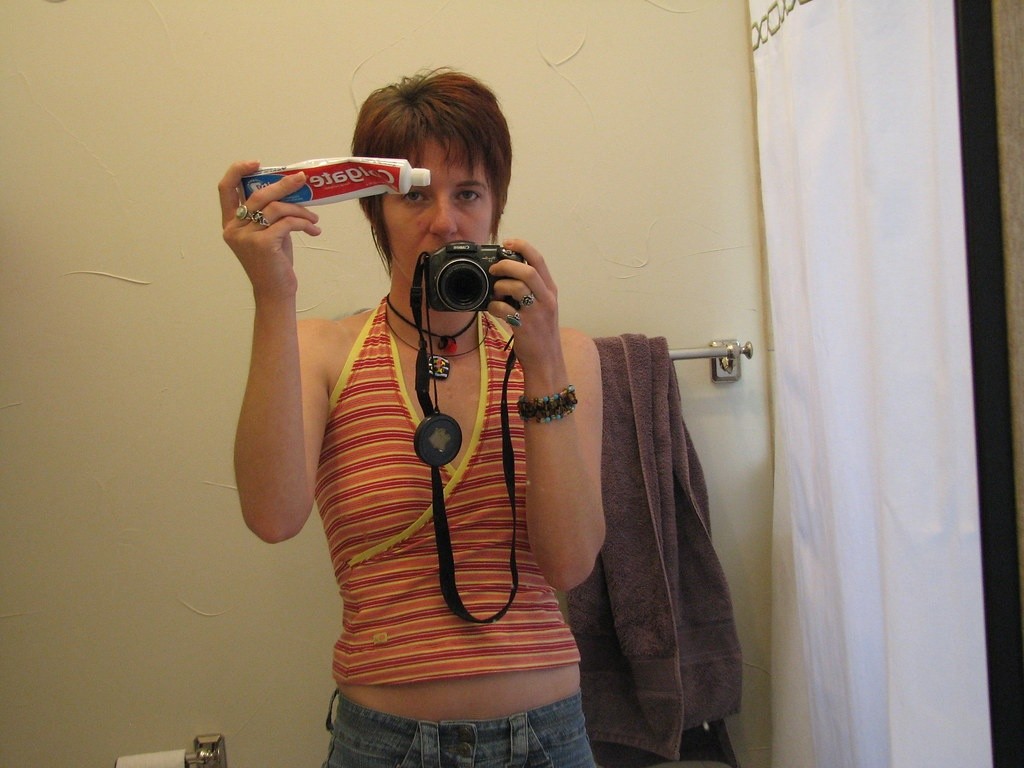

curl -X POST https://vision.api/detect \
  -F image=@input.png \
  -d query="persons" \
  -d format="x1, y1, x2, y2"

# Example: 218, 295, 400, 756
218, 66, 606, 768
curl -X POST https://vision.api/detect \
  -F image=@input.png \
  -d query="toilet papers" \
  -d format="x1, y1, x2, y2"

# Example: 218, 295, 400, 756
115, 749, 186, 768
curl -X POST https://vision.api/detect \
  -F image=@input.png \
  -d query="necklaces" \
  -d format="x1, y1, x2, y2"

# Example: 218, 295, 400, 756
387, 293, 478, 355
385, 303, 488, 379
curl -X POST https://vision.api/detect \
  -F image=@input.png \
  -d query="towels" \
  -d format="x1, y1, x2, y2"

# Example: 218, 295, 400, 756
569, 328, 748, 764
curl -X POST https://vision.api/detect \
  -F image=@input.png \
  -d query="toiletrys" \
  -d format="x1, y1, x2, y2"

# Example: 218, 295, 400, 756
245, 154, 436, 214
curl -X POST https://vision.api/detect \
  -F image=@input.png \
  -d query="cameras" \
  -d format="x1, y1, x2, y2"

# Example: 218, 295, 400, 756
425, 240, 525, 314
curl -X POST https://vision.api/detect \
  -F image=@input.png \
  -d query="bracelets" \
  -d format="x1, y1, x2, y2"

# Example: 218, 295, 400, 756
516, 384, 578, 423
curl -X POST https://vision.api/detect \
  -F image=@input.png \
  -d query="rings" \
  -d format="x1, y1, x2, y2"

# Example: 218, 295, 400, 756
236, 204, 252, 220
505, 311, 521, 327
519, 293, 535, 306
251, 209, 270, 226
